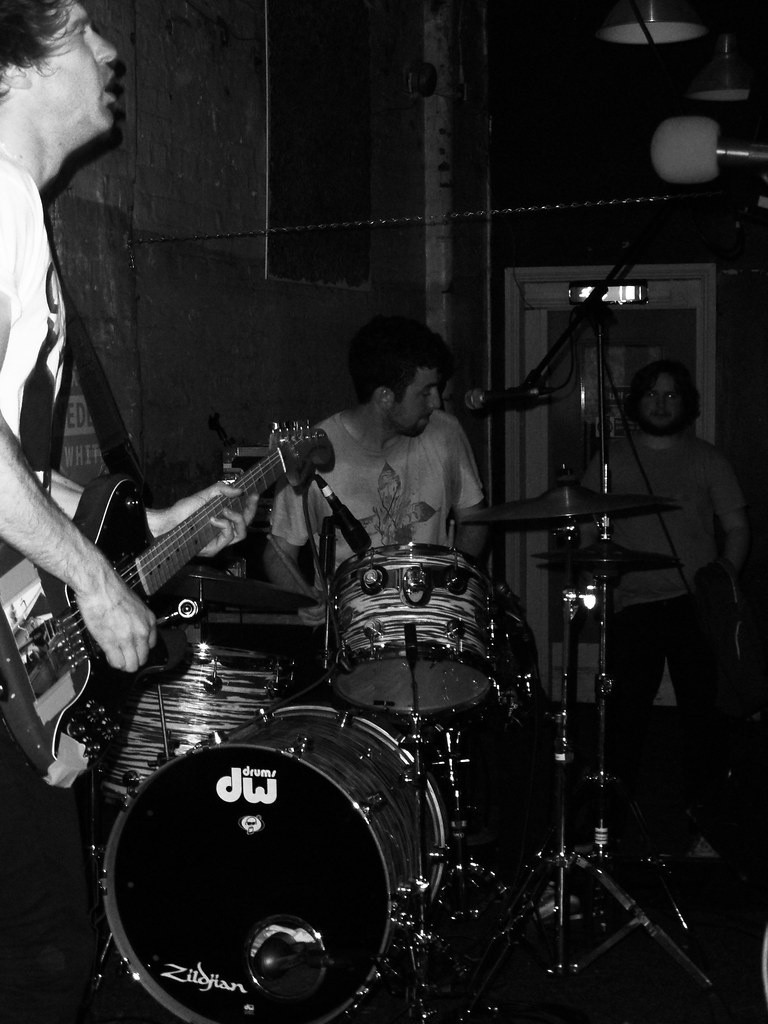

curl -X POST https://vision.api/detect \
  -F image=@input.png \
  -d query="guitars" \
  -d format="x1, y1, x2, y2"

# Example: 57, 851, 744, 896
0, 416, 335, 789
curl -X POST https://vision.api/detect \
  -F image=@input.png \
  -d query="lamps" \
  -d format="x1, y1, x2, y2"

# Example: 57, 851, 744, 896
688, 33, 750, 101
595, 0, 710, 46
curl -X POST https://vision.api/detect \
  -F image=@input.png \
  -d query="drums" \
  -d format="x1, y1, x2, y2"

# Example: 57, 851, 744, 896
328, 540, 495, 718
105, 705, 448, 1022
88, 576, 330, 807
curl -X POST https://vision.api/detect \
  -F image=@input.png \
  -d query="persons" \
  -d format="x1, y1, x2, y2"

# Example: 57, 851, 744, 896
579, 359, 749, 832
0, 1, 259, 1023
262, 315, 486, 592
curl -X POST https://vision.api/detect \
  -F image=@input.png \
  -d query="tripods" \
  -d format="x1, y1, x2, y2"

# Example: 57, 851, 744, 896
460, 254, 735, 1024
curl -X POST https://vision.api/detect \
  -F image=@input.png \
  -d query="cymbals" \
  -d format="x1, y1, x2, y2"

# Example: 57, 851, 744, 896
457, 482, 679, 524
530, 539, 684, 574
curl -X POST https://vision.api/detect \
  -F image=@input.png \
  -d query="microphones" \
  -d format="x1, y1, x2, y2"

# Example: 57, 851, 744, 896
464, 388, 548, 409
315, 473, 371, 555
